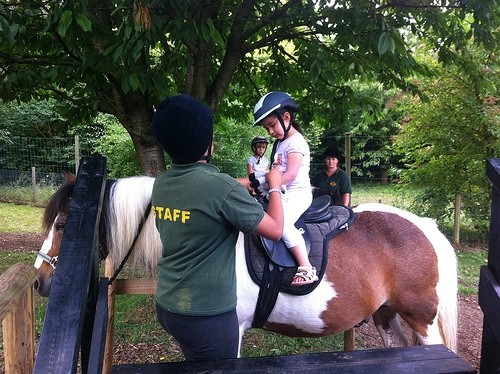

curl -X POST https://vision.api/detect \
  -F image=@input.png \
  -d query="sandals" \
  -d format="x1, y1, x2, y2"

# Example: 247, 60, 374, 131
292, 265, 319, 286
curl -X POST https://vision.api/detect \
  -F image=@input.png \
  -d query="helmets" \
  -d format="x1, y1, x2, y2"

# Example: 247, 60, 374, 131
322, 147, 340, 159
154, 93, 213, 163
251, 136, 269, 154
250, 91, 299, 127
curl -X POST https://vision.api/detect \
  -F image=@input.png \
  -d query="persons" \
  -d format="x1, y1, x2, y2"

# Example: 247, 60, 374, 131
152, 95, 284, 361
253, 91, 319, 285
248, 136, 352, 207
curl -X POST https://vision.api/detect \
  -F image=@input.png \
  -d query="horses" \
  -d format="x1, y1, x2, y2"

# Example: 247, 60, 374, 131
33, 176, 458, 355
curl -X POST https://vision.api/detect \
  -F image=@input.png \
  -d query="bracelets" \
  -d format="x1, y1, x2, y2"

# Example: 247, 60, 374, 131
268, 189, 282, 194
